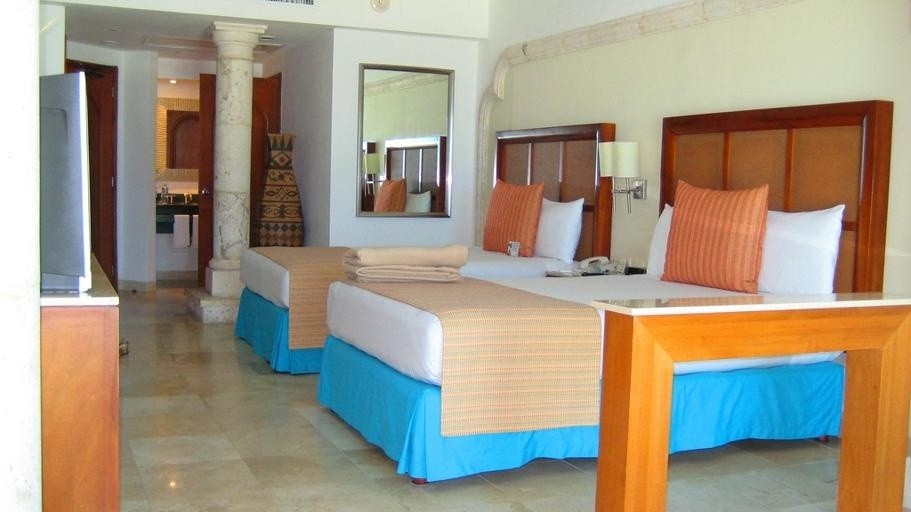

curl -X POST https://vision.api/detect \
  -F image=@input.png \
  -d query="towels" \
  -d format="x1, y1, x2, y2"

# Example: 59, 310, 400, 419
193, 215, 198, 249
174, 215, 190, 248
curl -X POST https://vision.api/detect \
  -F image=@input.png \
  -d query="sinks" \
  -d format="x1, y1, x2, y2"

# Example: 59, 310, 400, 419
156, 201, 199, 207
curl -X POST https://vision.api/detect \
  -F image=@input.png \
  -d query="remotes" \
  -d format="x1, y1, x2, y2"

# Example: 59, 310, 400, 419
545, 270, 581, 277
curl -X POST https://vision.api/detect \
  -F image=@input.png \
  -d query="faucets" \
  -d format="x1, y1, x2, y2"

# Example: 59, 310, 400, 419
165, 194, 176, 202
183, 192, 192, 203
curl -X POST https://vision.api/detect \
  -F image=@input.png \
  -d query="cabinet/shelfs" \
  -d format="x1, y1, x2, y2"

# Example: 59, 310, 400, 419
41, 251, 121, 511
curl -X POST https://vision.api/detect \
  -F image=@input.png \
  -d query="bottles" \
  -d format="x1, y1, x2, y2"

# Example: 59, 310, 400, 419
162, 183, 168, 194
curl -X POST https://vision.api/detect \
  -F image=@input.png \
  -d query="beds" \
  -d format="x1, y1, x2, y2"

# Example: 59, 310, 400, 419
317, 101, 894, 484
238, 124, 615, 372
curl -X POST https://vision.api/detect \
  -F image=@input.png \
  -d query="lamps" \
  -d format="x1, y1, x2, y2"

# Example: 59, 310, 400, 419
600, 142, 648, 213
365, 154, 384, 184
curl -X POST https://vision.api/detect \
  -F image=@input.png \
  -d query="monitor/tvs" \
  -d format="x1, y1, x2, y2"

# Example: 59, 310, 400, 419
39, 71, 92, 292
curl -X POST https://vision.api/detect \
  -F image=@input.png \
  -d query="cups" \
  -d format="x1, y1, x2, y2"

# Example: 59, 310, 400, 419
184, 193, 193, 205
162, 195, 177, 204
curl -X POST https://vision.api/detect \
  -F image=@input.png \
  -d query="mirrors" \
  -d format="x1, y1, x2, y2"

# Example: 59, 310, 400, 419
356, 63, 456, 218
157, 99, 200, 181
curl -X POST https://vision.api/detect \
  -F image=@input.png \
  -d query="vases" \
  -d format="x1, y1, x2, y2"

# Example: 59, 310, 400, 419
254, 134, 305, 246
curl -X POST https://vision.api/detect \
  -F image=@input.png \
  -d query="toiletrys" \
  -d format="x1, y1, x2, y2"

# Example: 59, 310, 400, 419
162, 182, 168, 195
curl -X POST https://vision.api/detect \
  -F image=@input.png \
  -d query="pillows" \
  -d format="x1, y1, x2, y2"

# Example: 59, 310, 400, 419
406, 192, 432, 212
375, 179, 408, 212
537, 197, 585, 262
649, 180, 845, 295
483, 179, 545, 257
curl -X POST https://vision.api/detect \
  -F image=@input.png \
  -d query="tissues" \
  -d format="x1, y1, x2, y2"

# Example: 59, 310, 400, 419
624, 251, 646, 274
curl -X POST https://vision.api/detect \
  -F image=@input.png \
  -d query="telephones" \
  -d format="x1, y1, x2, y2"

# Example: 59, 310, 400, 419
573, 256, 610, 272
